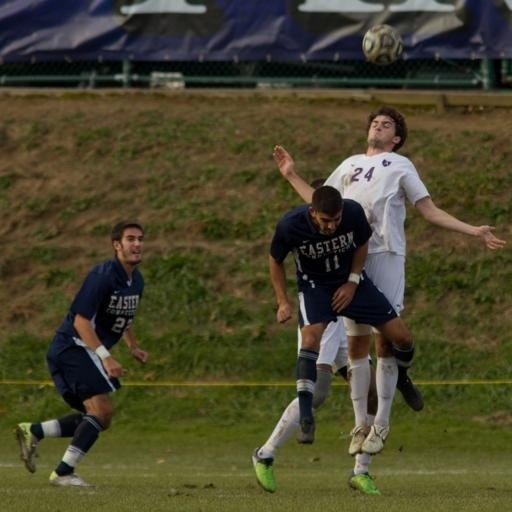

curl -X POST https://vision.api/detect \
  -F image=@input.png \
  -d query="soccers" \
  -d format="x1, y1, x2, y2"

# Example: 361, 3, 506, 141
363, 25, 403, 65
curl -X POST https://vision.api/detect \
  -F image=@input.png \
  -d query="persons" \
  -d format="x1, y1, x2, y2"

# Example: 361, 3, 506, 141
252, 178, 378, 494
16, 220, 148, 489
270, 186, 425, 444
273, 107, 506, 457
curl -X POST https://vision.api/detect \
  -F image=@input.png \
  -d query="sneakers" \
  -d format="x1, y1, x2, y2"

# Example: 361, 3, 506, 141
13, 421, 38, 475
394, 370, 425, 412
295, 417, 317, 444
47, 469, 96, 489
347, 424, 370, 457
250, 447, 276, 494
347, 470, 382, 497
360, 419, 391, 457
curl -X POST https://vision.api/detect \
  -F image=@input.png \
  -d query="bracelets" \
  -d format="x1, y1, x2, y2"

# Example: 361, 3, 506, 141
347, 272, 360, 285
94, 344, 110, 361
